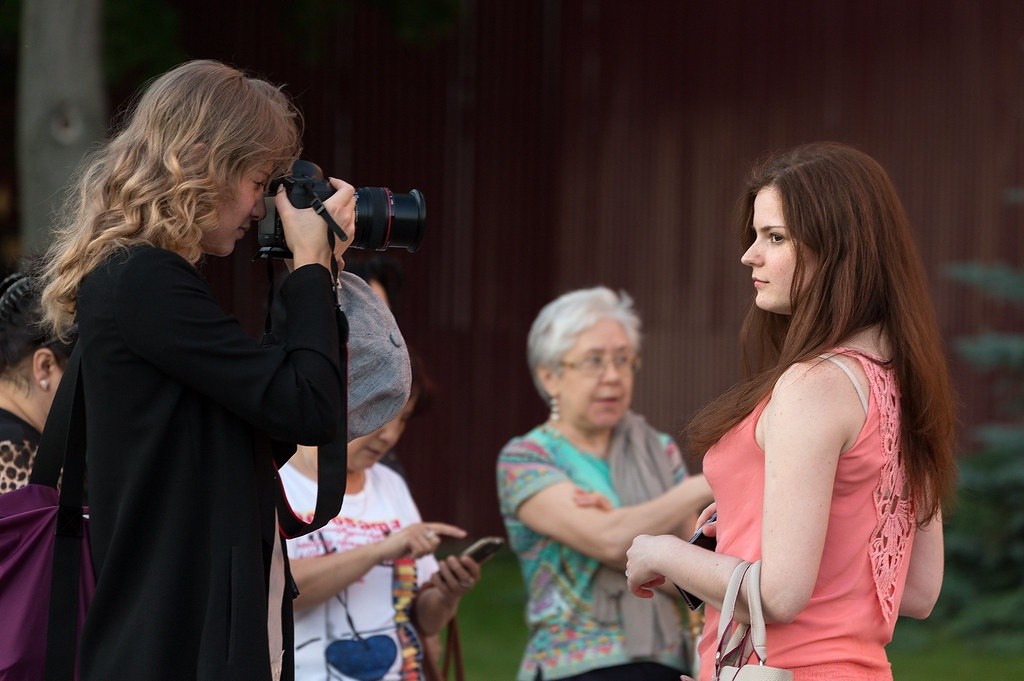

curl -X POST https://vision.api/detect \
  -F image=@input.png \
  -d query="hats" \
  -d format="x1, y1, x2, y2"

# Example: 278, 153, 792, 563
325, 267, 412, 441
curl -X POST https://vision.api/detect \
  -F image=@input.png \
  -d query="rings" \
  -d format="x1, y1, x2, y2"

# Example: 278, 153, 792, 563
625, 569, 629, 578
425, 532, 435, 540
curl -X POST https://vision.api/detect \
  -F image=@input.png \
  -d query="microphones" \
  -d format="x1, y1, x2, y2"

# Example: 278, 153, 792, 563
673, 512, 716, 612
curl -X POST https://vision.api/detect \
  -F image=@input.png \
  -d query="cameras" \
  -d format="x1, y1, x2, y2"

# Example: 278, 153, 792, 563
257, 161, 424, 257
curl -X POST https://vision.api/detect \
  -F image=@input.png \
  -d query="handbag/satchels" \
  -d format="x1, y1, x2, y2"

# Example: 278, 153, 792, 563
679, 562, 794, 681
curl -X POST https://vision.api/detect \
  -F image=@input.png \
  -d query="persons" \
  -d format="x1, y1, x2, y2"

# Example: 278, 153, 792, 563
1, 265, 94, 681
40, 61, 358, 681
277, 348, 496, 680
497, 284, 715, 680
624, 144, 965, 681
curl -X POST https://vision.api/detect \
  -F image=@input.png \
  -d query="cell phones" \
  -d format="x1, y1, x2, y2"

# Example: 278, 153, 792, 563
431, 537, 504, 584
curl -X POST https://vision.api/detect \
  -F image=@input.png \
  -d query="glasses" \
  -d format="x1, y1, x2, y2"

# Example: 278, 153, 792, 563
560, 353, 644, 378
